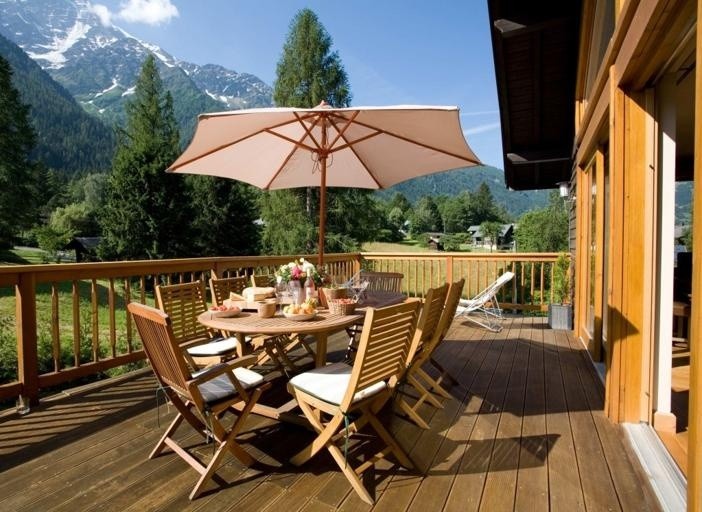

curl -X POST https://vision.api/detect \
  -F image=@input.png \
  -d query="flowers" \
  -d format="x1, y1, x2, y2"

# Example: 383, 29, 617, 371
275, 259, 332, 288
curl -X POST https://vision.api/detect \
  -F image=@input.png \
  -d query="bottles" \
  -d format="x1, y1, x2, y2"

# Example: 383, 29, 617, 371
303, 267, 316, 301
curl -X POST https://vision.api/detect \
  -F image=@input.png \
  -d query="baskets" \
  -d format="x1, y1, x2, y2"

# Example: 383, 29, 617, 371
317, 269, 406, 317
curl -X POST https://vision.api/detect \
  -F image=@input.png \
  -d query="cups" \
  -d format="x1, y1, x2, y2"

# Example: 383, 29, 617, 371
257, 302, 276, 318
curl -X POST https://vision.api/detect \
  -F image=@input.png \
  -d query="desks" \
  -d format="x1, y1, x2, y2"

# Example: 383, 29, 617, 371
197, 286, 407, 433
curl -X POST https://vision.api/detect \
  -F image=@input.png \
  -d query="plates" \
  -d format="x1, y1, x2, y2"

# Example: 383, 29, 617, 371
207, 308, 242, 317
282, 310, 318, 321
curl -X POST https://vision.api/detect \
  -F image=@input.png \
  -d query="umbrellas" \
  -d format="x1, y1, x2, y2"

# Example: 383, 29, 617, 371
164, 97, 487, 288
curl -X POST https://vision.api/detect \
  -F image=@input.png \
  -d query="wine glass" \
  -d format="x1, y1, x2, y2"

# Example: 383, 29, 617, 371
275, 284, 287, 318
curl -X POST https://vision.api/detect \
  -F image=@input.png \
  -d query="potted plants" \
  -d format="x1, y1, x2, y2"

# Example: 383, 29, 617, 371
548, 253, 573, 331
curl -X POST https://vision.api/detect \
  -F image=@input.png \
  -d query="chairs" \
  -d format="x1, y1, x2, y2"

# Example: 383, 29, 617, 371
390, 282, 445, 432
254, 276, 315, 366
453, 271, 515, 332
343, 272, 405, 358
209, 278, 290, 384
424, 277, 465, 401
286, 298, 423, 505
127, 302, 273, 501
152, 279, 214, 358
